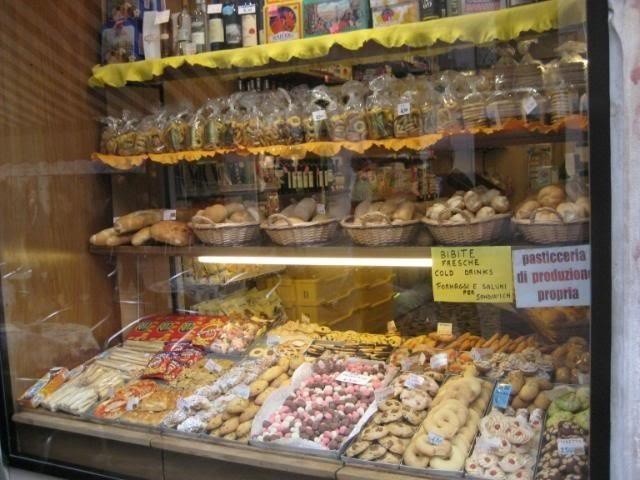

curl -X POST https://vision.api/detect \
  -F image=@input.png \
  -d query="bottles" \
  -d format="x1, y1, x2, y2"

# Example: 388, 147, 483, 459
207, 0, 224, 50
192, 0, 205, 52
241, 0, 259, 47
222, 0, 241, 49
159, 17, 173, 58
176, 0, 190, 55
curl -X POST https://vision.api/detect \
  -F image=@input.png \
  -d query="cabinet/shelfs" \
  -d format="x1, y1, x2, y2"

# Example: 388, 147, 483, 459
1, 0, 591, 476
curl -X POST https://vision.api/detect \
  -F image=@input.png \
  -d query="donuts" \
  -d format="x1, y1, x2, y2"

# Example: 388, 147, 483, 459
403, 377, 493, 471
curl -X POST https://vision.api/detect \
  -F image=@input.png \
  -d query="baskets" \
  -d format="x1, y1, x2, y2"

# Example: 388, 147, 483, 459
260, 214, 341, 246
510, 206, 590, 246
526, 306, 590, 344
421, 207, 513, 246
187, 216, 262, 246
339, 210, 425, 247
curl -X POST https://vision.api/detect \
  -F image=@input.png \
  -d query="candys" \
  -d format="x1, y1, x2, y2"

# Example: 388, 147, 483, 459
250, 357, 387, 453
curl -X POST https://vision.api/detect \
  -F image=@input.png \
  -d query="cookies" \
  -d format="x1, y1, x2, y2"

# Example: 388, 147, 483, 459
99, 63, 590, 156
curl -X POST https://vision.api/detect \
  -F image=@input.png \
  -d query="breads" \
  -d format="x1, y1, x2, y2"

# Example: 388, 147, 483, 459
272, 195, 330, 226
193, 200, 254, 226
354, 192, 422, 227
533, 389, 591, 479
516, 177, 590, 223
93, 355, 234, 427
207, 355, 307, 443
464, 407, 543, 480
89, 204, 196, 248
427, 186, 508, 223
346, 371, 445, 465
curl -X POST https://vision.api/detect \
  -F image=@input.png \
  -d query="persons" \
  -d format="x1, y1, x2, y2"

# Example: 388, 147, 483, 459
430, 143, 481, 335
350, 155, 385, 208
